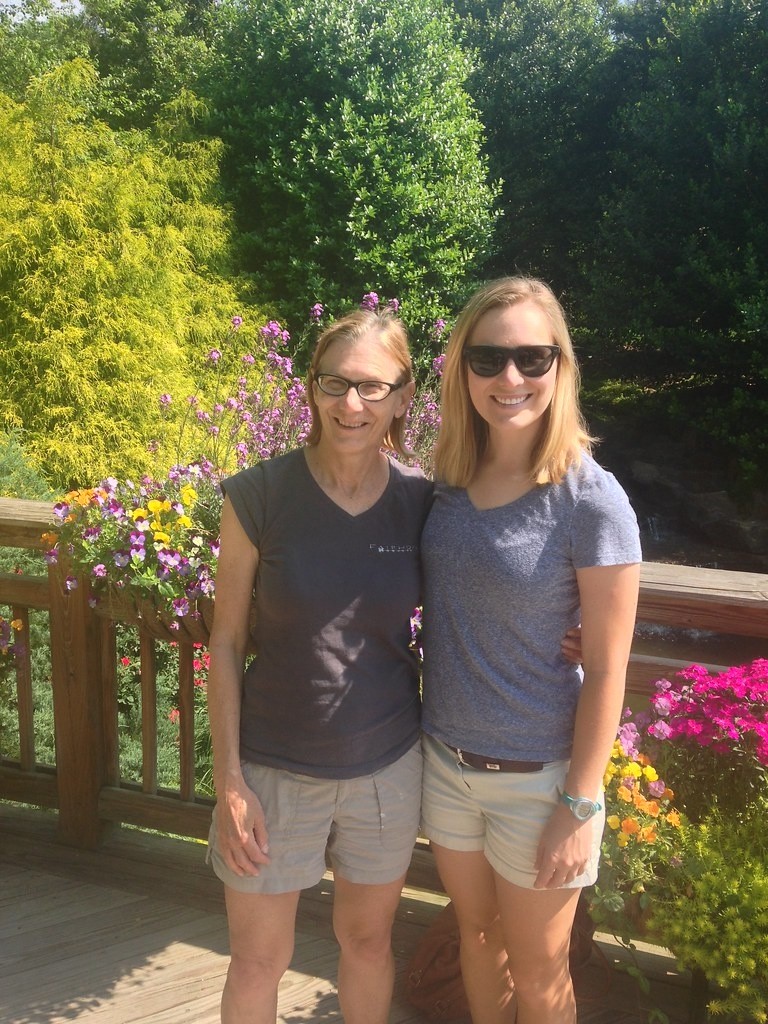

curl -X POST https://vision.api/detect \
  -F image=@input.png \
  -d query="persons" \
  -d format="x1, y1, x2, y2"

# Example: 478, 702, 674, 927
206, 310, 584, 1024
420, 278, 642, 1024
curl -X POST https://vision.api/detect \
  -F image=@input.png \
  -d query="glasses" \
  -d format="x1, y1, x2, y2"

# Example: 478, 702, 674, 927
313, 371, 403, 402
462, 344, 561, 377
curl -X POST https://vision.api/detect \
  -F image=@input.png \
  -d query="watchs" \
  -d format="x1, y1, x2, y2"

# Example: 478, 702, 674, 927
562, 791, 602, 821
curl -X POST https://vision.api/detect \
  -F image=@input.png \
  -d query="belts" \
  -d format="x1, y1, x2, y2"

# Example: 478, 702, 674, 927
444, 743, 552, 773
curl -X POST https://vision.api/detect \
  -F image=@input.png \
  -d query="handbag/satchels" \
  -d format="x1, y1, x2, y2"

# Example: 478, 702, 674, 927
401, 894, 610, 1024
